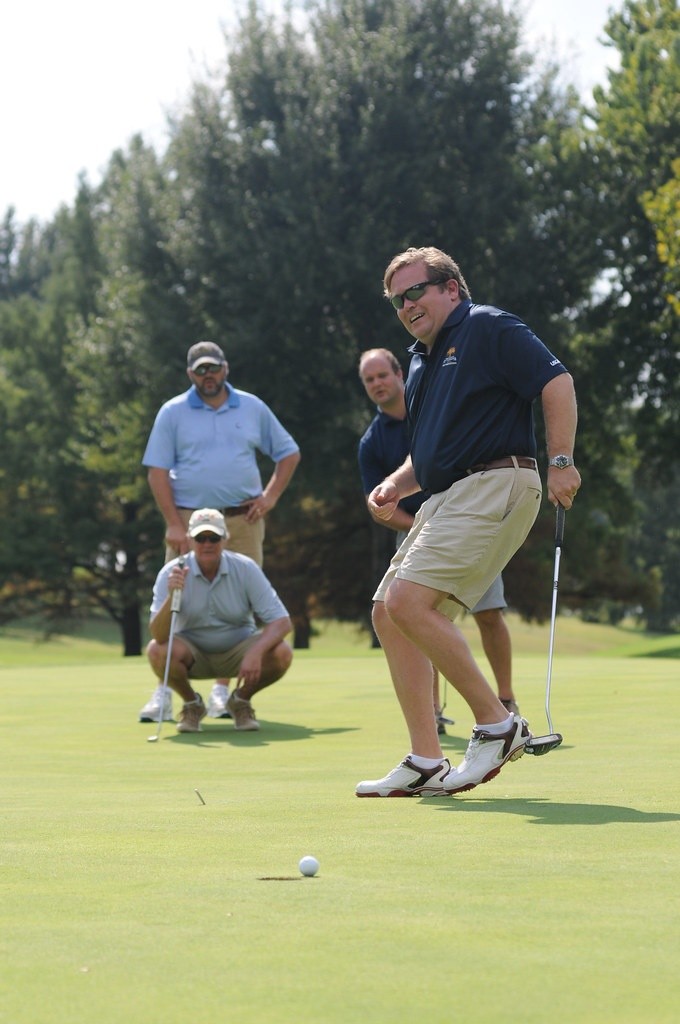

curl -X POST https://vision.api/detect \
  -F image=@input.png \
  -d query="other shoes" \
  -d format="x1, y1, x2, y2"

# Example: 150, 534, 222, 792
433, 714, 445, 734
498, 697, 520, 718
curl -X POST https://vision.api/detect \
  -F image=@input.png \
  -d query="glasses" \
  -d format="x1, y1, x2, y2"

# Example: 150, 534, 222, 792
192, 363, 223, 375
390, 278, 447, 309
193, 534, 222, 544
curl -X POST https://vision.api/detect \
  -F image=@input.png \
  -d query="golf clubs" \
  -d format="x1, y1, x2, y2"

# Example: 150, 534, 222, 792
436, 678, 456, 725
147, 559, 185, 743
523, 502, 569, 757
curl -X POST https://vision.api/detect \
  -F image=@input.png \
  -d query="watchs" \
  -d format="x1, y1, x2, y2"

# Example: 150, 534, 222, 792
549, 456, 574, 468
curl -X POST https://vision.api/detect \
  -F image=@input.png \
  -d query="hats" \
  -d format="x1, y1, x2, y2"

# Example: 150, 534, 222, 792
186, 508, 230, 539
187, 340, 226, 372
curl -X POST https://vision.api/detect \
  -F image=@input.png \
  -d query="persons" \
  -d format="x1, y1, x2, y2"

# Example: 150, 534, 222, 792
140, 341, 301, 721
358, 347, 520, 732
147, 508, 292, 731
355, 247, 580, 793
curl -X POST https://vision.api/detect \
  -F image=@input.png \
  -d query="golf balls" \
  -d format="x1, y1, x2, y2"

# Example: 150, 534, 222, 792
298, 857, 319, 877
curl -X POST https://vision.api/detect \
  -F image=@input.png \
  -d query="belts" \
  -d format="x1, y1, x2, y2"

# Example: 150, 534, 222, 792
176, 505, 253, 517
459, 456, 536, 479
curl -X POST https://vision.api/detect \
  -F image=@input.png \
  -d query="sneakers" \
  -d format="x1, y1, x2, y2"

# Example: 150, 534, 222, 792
442, 713, 533, 794
355, 755, 455, 797
176, 693, 208, 732
139, 691, 172, 721
207, 686, 230, 719
224, 689, 259, 731
